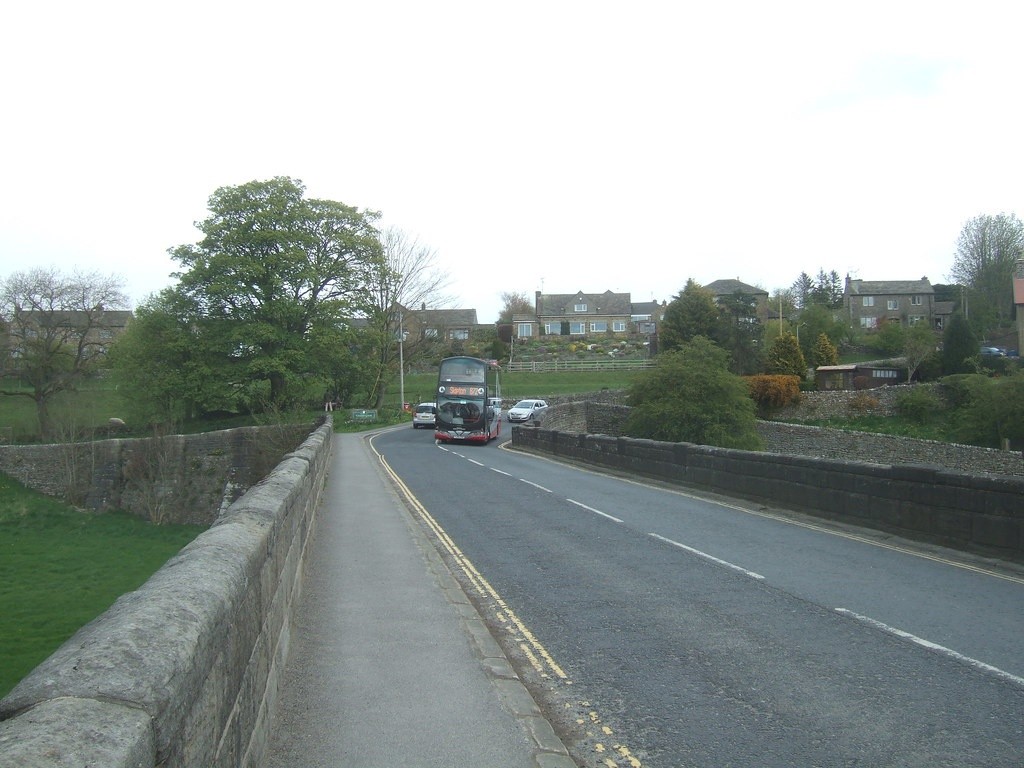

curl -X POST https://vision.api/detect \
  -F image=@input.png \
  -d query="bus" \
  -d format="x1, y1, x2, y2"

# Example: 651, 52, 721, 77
433, 356, 504, 443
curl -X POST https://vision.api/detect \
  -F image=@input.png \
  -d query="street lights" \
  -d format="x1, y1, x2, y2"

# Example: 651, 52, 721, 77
776, 293, 783, 336
400, 301, 426, 410
796, 323, 807, 346
510, 339, 516, 364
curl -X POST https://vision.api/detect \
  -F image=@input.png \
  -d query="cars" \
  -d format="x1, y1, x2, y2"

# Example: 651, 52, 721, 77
507, 399, 548, 422
981, 346, 1020, 357
487, 398, 501, 420
412, 402, 437, 429
